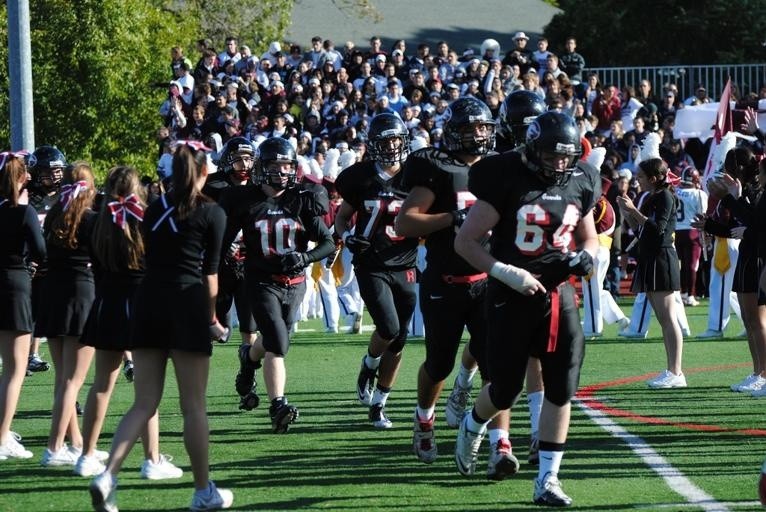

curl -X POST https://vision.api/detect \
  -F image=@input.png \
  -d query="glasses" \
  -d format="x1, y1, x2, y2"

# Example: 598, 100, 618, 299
516, 38, 523, 41
639, 84, 647, 88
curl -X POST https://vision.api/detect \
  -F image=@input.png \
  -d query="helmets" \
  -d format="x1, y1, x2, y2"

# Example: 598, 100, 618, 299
219, 136, 257, 177
26, 146, 68, 192
679, 165, 700, 186
441, 97, 496, 156
498, 90, 550, 147
365, 113, 410, 165
250, 137, 299, 188
524, 111, 583, 187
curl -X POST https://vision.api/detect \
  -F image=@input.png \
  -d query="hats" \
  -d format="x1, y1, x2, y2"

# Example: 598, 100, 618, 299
392, 49, 404, 57
646, 102, 658, 114
430, 127, 443, 134
446, 83, 459, 89
375, 54, 386, 62
270, 41, 281, 54
696, 83, 706, 92
429, 91, 440, 98
601, 176, 612, 195
511, 32, 530, 41
664, 91, 674, 97
430, 78, 441, 83
412, 103, 422, 112
170, 79, 183, 95
300, 131, 313, 144
575, 82, 589, 98
240, 44, 250, 53
223, 118, 241, 130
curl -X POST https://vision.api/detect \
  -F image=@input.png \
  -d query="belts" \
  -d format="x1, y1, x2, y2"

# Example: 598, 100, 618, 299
442, 272, 488, 284
271, 273, 305, 287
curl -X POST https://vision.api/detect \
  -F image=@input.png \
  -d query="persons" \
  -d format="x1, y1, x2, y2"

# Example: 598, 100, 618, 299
616, 158, 688, 388
226, 138, 335, 435
585, 73, 765, 147
123, 349, 134, 383
610, 176, 630, 280
581, 145, 631, 340
41, 162, 110, 469
690, 130, 749, 339
75, 165, 183, 482
617, 134, 690, 341
155, 31, 586, 153
286, 146, 366, 337
216, 137, 258, 347
673, 166, 707, 307
445, 90, 549, 466
333, 114, 421, 430
722, 158, 766, 399
407, 135, 428, 338
394, 96, 498, 465
26, 146, 68, 376
87, 140, 233, 512
0, 152, 45, 460
454, 111, 600, 508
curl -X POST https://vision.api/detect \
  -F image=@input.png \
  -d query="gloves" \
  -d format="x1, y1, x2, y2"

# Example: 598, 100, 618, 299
559, 249, 594, 278
280, 251, 310, 274
490, 259, 540, 292
226, 243, 240, 258
344, 235, 371, 257
451, 206, 471, 228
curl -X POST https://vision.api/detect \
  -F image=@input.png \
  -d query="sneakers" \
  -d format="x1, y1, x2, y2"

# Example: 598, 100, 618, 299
140, 455, 183, 480
41, 443, 77, 467
687, 299, 699, 306
349, 310, 363, 334
682, 328, 691, 337
73, 454, 107, 477
412, 406, 438, 464
324, 326, 340, 334
368, 403, 392, 428
445, 373, 473, 429
750, 383, 766, 397
88, 471, 119, 512
527, 437, 540, 465
234, 342, 263, 397
269, 397, 300, 434
454, 413, 487, 476
648, 370, 688, 388
736, 328, 748, 338
238, 391, 260, 411
356, 354, 378, 406
26, 353, 51, 372
623, 330, 648, 338
69, 444, 110, 461
617, 317, 631, 336
730, 374, 756, 392
533, 475, 573, 507
0, 432, 34, 461
737, 375, 766, 392
122, 363, 135, 382
487, 437, 520, 480
190, 480, 234, 511
695, 328, 724, 339
